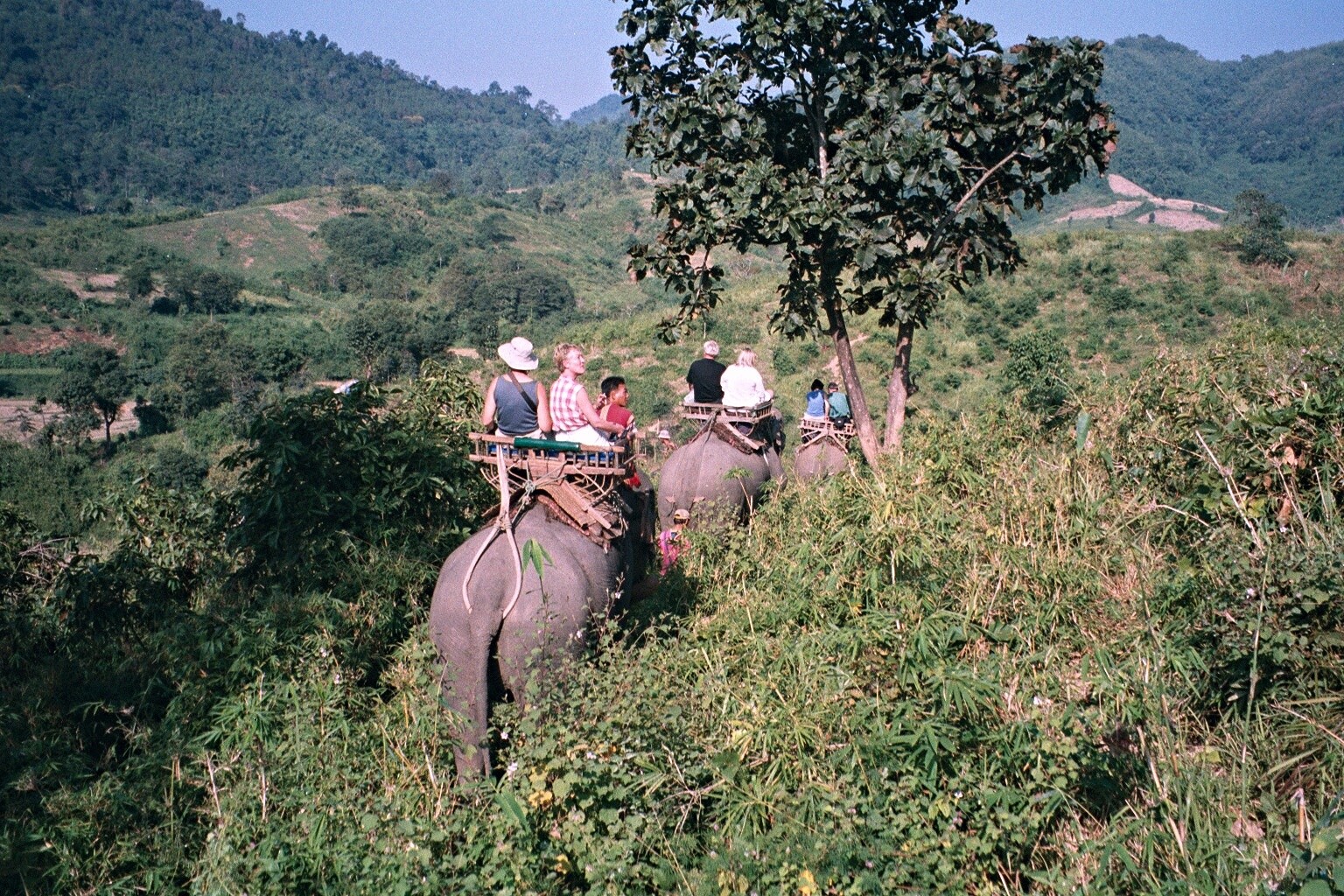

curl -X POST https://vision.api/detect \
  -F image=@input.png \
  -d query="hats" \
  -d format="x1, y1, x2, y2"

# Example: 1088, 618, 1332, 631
658, 430, 671, 439
674, 509, 694, 522
498, 337, 539, 370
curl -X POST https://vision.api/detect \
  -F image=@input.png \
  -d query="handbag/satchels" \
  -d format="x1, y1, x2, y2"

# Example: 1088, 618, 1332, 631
835, 418, 845, 428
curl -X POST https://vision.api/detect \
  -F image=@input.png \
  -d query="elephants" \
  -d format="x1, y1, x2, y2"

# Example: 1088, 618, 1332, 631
657, 429, 787, 575
430, 467, 656, 797
795, 431, 860, 492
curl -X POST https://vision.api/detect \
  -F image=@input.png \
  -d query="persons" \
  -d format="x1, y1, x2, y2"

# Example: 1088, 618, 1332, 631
549, 343, 630, 455
483, 338, 552, 451
596, 376, 637, 449
804, 379, 829, 421
827, 382, 853, 424
720, 348, 774, 416
687, 340, 726, 411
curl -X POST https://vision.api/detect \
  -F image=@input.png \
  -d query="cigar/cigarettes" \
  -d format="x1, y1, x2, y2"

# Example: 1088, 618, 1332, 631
604, 393, 605, 397
